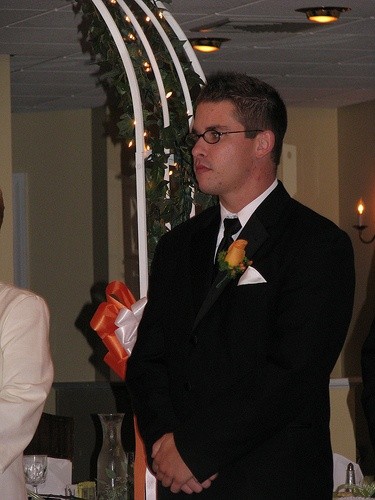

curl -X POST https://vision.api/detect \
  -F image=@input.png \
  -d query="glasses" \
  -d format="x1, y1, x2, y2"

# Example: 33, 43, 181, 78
181, 131, 258, 148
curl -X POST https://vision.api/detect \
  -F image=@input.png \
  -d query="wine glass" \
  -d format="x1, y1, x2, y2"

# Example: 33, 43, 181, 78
23, 455, 47, 500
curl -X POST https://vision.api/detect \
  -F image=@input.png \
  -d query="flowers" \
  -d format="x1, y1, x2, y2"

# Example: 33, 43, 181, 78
216, 240, 254, 288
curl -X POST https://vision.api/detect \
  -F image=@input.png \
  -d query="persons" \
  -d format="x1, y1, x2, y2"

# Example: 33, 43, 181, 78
0, 187, 54, 500
128, 72, 356, 500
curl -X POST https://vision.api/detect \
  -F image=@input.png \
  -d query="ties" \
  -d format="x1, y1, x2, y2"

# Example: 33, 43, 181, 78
215, 217, 242, 269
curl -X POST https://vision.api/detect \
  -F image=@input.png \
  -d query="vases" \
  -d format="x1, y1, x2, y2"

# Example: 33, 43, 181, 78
97, 412, 128, 500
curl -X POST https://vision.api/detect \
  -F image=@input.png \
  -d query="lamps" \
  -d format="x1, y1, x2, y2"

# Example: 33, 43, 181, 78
352, 200, 375, 244
296, 6, 351, 23
189, 37, 229, 52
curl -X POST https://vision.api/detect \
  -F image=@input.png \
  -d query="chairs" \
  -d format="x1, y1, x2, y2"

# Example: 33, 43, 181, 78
23, 412, 90, 484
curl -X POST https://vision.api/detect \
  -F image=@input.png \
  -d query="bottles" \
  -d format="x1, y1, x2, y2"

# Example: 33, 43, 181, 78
96, 411, 129, 500
346, 463, 355, 492
